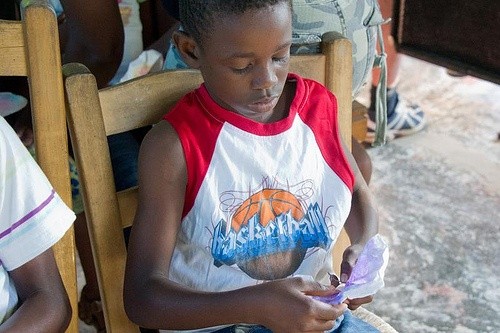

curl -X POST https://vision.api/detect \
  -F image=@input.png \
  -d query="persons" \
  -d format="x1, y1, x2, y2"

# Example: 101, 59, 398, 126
0, 0, 426, 333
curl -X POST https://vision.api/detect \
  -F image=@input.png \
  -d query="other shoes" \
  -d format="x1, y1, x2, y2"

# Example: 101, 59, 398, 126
366, 95, 428, 143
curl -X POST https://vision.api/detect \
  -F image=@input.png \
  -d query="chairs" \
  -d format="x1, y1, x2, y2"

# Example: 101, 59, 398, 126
0, 1, 79, 333
63, 31, 353, 332
363, 0, 500, 146
